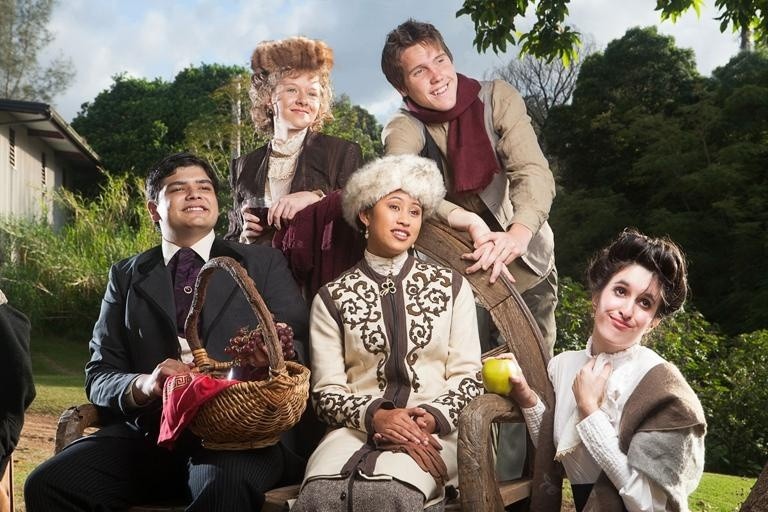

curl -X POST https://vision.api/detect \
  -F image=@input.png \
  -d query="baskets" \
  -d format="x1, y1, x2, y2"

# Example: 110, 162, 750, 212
184, 256, 312, 451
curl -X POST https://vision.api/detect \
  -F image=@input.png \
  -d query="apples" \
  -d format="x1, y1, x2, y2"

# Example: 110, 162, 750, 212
481, 357, 517, 396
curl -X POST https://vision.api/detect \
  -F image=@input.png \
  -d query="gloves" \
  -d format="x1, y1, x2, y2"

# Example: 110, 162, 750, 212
379, 429, 450, 485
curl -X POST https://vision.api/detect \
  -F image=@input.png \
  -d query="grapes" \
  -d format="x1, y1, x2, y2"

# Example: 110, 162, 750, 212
222, 322, 295, 368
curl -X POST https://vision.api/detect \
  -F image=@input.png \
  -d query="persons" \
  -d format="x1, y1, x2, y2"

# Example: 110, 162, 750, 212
0, 289, 36, 512
380, 17, 560, 359
222, 35, 363, 248
473, 228, 708, 512
24, 153, 309, 512
286, 151, 496, 512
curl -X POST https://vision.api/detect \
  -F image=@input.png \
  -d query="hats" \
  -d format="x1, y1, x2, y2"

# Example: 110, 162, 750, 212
341, 154, 447, 234
251, 36, 333, 74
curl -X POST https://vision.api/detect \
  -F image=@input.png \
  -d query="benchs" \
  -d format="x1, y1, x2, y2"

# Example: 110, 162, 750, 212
56, 218, 562, 512
